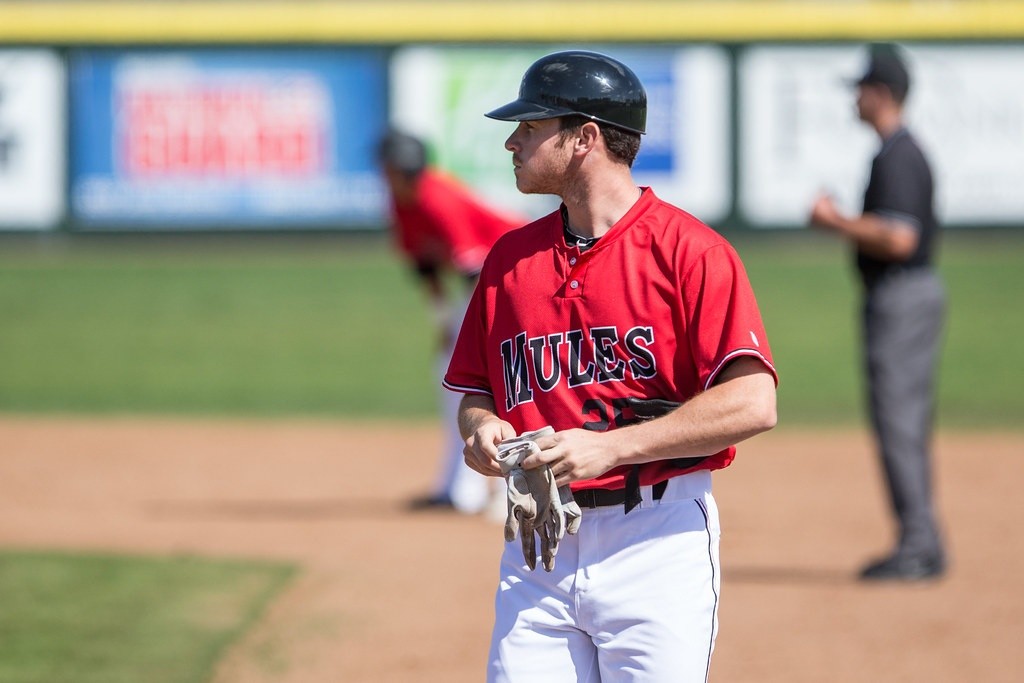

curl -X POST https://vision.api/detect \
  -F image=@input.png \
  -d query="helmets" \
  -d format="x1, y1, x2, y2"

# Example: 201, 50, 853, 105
484, 50, 646, 135
381, 134, 426, 176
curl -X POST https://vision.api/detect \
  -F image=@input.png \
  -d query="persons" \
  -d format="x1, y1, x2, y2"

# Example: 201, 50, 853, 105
440, 49, 777, 683
362, 116, 528, 516
810, 40, 951, 584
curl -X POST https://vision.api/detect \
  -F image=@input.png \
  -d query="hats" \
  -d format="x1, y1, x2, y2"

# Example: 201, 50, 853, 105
855, 44, 909, 101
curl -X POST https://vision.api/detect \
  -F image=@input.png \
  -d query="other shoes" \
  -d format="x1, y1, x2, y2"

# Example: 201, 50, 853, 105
861, 547, 946, 578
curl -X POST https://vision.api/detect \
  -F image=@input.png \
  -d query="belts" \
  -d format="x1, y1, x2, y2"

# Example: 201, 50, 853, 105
573, 488, 625, 509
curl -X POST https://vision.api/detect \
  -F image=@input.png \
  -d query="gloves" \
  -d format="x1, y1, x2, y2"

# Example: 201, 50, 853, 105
501, 425, 582, 536
495, 446, 566, 572
